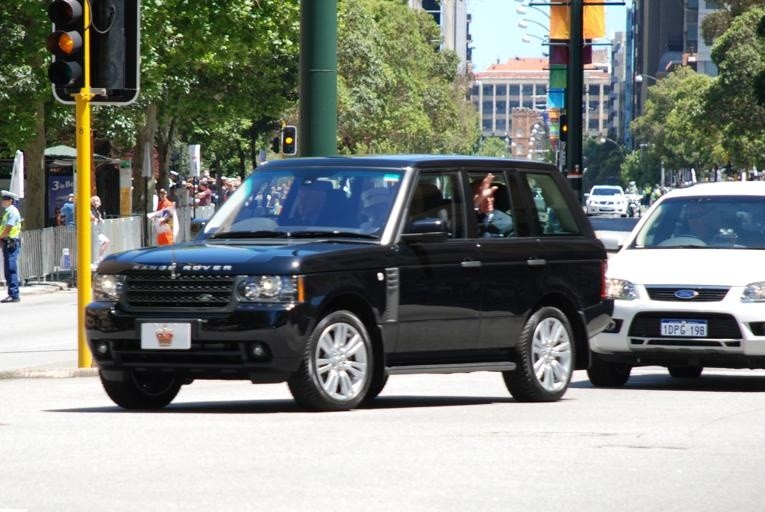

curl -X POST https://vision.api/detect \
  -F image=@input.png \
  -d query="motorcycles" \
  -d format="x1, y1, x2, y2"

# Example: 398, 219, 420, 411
624, 186, 644, 218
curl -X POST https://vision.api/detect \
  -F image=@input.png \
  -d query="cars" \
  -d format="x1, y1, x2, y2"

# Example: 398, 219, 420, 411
587, 181, 765, 388
584, 185, 630, 218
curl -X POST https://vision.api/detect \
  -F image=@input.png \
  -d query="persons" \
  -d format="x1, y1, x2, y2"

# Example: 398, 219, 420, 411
48, 207, 64, 227
470, 172, 513, 237
91, 195, 109, 264
58, 194, 75, 247
682, 203, 716, 241
0, 190, 21, 302
627, 180, 669, 220
130, 169, 293, 245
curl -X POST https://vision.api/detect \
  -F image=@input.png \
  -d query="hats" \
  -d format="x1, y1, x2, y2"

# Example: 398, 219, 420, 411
1, 190, 19, 202
360, 186, 392, 208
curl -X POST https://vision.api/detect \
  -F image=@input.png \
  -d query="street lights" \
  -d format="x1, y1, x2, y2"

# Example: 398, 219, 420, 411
600, 136, 625, 160
514, 0, 551, 44
524, 123, 547, 160
635, 74, 659, 84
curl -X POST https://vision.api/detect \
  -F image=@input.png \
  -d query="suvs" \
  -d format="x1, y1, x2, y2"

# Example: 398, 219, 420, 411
84, 154, 615, 412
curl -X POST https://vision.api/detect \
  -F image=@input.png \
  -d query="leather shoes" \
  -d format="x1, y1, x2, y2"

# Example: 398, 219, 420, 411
0, 295, 19, 302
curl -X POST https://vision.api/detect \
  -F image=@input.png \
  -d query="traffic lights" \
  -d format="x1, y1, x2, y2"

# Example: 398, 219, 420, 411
559, 115, 568, 142
281, 125, 297, 155
269, 136, 279, 154
47, 1, 85, 102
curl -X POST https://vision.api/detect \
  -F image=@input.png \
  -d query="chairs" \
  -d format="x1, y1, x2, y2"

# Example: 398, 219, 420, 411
280, 179, 333, 227
